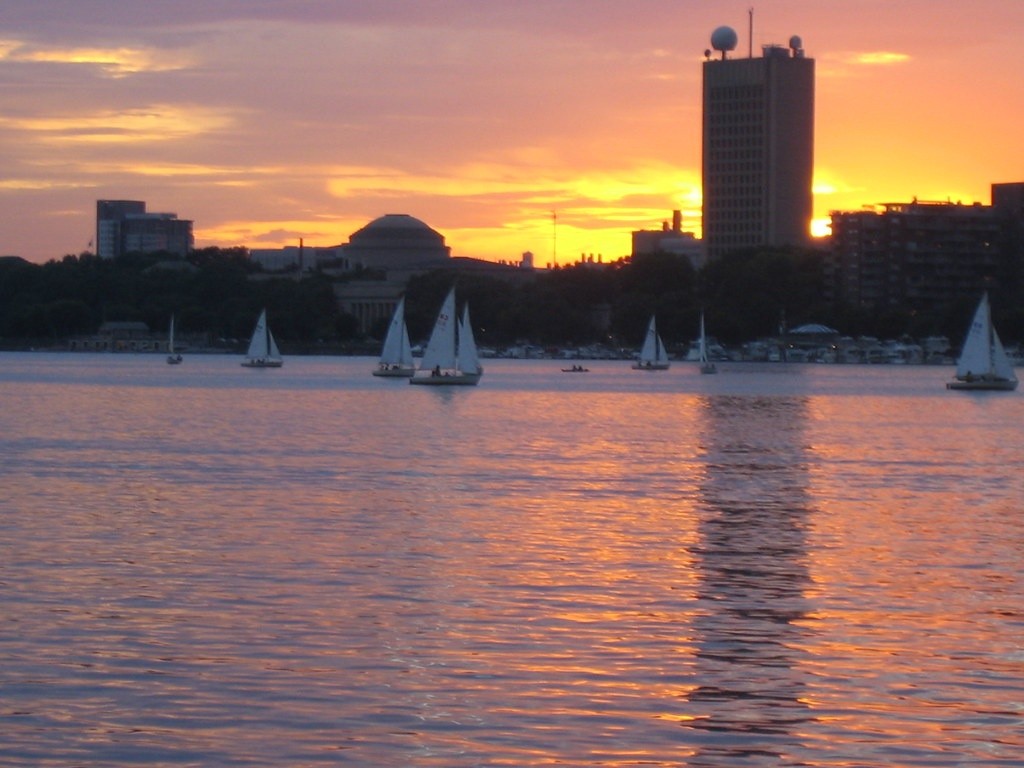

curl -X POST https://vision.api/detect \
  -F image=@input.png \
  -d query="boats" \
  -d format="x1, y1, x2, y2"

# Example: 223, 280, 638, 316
560, 366, 589, 373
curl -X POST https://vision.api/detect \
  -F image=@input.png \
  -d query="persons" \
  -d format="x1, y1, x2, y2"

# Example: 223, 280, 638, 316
966, 371, 974, 383
431, 365, 450, 377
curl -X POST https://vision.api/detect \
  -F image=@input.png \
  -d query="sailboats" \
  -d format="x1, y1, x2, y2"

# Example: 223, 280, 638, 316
166, 314, 184, 365
945, 288, 1020, 390
409, 285, 485, 386
632, 313, 671, 370
700, 312, 717, 374
240, 307, 287, 368
371, 293, 418, 378
